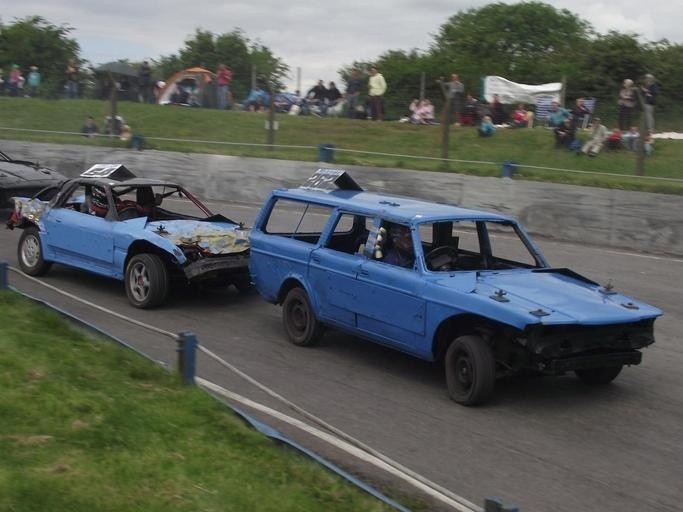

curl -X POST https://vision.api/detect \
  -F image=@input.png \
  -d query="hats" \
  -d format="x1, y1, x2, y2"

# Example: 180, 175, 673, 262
552, 97, 561, 105
594, 117, 600, 121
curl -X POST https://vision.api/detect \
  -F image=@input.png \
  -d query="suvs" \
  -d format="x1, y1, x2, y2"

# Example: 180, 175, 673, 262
246, 169, 665, 408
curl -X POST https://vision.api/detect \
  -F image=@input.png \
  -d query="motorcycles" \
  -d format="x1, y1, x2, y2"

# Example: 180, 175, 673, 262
288, 95, 350, 116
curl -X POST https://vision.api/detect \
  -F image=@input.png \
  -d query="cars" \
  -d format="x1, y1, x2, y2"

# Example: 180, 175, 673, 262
5, 163, 256, 311
0, 152, 74, 218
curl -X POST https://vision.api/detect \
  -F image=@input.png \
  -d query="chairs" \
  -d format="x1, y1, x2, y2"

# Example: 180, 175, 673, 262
137, 185, 157, 212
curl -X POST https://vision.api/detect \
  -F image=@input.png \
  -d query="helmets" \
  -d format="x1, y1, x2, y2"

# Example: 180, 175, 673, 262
390, 224, 411, 237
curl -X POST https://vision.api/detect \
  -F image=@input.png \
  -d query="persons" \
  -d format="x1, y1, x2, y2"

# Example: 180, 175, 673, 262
85, 186, 145, 219
444, 71, 661, 159
136, 61, 152, 104
79, 113, 146, 151
236, 68, 388, 123
208, 61, 232, 111
381, 223, 416, 270
397, 96, 436, 127
0, 58, 83, 101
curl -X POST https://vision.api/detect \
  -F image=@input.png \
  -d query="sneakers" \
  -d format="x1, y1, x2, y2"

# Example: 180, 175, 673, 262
575, 149, 599, 159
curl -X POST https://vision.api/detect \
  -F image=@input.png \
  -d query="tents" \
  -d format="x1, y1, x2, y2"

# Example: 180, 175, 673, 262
157, 66, 234, 110
